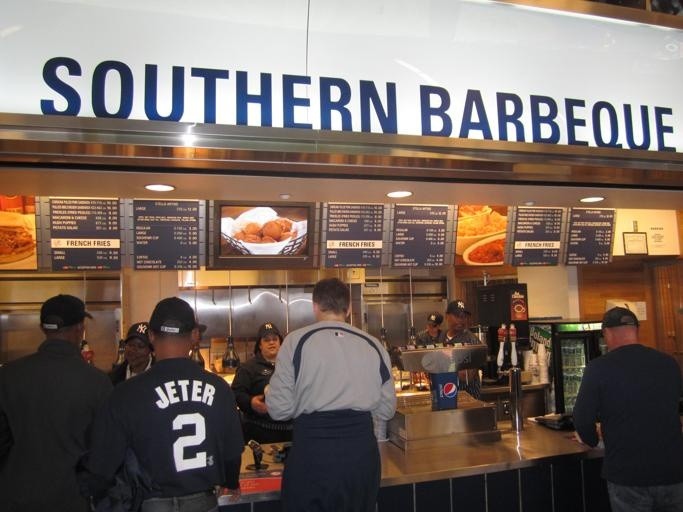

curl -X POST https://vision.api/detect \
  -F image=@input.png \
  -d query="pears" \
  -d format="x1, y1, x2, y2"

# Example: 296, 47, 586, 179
234, 219, 295, 244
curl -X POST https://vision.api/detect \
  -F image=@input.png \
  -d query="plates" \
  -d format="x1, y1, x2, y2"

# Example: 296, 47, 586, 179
461, 234, 507, 265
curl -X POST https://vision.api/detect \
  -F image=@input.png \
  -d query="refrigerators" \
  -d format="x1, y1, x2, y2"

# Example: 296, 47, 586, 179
528, 317, 608, 416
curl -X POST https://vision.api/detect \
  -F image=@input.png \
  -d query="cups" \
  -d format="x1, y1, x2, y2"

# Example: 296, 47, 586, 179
523, 343, 548, 386
428, 372, 459, 411
514, 305, 525, 318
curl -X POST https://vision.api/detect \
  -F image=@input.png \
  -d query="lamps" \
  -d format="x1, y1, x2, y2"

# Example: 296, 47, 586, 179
79, 271, 90, 359
111, 272, 127, 366
378, 265, 393, 353
221, 269, 240, 369
189, 287, 204, 369
406, 266, 421, 347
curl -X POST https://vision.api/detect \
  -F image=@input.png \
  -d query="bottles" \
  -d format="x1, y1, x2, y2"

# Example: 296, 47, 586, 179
221, 336, 240, 369
561, 339, 583, 394
114, 338, 125, 364
564, 394, 577, 413
379, 329, 389, 351
406, 328, 416, 346
79, 344, 93, 365
190, 345, 205, 369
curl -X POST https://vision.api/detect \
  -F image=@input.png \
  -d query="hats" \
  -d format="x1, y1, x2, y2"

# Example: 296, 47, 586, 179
426, 312, 443, 325
602, 307, 638, 327
40, 294, 92, 328
127, 298, 208, 347
258, 322, 279, 336
447, 300, 470, 315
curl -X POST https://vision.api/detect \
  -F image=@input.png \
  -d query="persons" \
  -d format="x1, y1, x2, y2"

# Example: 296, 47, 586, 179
231, 321, 293, 445
1, 294, 114, 512
111, 322, 155, 387
443, 300, 482, 400
100, 296, 246, 512
571, 306, 683, 512
416, 312, 445, 348
264, 277, 397, 512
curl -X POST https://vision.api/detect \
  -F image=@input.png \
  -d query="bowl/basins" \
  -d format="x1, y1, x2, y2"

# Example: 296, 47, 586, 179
456, 216, 508, 254
